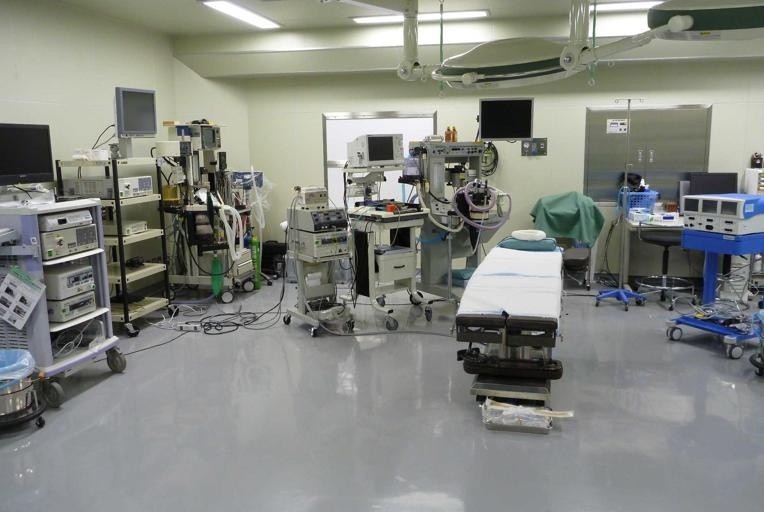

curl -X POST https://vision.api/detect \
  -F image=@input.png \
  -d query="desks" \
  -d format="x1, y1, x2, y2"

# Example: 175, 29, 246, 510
619, 213, 691, 293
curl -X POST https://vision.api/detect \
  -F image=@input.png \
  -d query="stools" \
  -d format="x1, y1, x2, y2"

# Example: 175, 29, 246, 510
635, 225, 700, 310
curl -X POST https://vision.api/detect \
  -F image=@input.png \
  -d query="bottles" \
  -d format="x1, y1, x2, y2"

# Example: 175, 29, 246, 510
452, 126, 458, 142
445, 126, 452, 142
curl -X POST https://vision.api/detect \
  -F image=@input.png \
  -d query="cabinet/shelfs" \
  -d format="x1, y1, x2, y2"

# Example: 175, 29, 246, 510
55, 154, 167, 328
0, 196, 118, 374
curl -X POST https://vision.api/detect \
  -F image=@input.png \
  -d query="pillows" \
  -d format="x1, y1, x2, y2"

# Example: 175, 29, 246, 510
513, 226, 545, 241
500, 234, 555, 254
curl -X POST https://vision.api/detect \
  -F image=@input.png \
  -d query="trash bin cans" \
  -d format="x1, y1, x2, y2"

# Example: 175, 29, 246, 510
0, 348, 38, 425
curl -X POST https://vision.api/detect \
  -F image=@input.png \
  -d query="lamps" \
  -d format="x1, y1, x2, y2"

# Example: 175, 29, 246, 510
433, 0, 764, 90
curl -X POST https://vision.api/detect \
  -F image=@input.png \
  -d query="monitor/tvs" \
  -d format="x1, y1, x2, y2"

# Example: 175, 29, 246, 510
480, 96, 534, 141
113, 85, 159, 140
0, 123, 56, 186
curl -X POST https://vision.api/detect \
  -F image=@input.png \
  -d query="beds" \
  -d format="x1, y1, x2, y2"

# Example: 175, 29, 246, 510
457, 236, 565, 414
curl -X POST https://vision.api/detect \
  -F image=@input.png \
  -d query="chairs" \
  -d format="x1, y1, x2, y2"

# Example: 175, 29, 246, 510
532, 194, 600, 290
